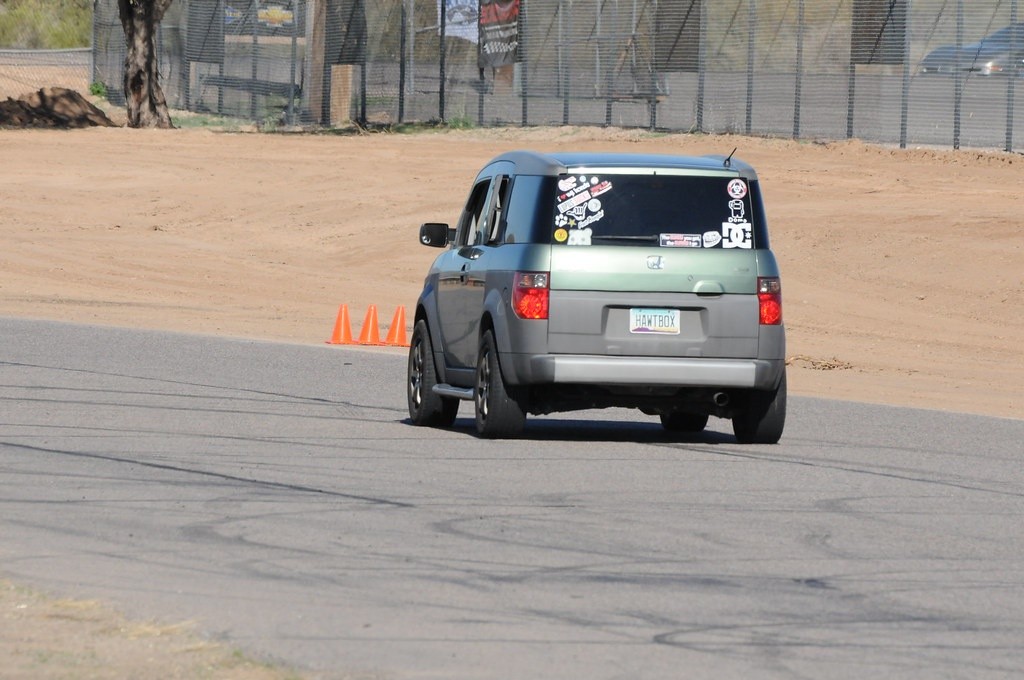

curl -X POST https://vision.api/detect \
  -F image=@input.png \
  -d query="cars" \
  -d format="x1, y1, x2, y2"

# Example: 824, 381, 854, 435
921, 23, 1024, 79
407, 150, 787, 444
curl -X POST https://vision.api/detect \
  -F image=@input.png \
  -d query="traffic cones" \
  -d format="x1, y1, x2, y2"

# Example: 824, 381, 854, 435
325, 304, 357, 345
382, 306, 412, 348
355, 306, 386, 347
